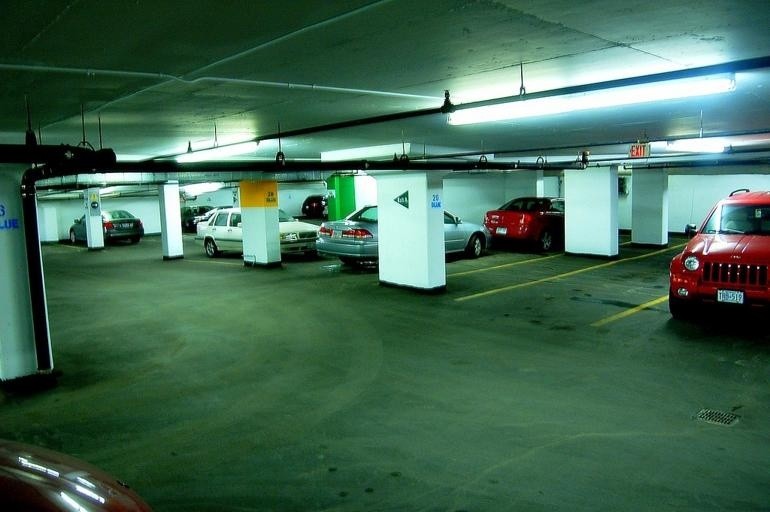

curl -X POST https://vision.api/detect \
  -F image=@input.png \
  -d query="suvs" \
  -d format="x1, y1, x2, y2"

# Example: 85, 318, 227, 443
670, 190, 770, 322
300, 193, 334, 216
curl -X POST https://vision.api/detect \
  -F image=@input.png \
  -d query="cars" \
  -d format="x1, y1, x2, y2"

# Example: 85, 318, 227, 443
181, 206, 213, 232
317, 206, 490, 266
1, 439, 153, 512
68, 209, 144, 245
195, 208, 320, 256
482, 197, 565, 254
